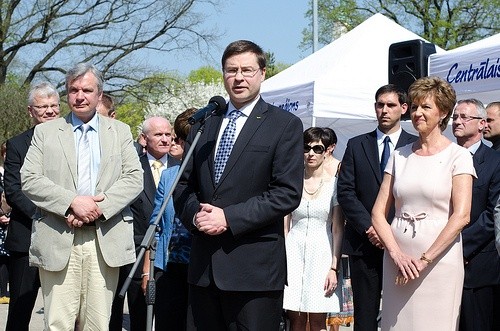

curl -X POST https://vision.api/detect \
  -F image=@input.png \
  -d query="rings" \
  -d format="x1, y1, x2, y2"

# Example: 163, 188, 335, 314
332, 287, 334, 289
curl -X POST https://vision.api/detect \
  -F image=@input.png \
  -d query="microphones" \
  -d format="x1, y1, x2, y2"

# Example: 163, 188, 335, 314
187, 96, 226, 125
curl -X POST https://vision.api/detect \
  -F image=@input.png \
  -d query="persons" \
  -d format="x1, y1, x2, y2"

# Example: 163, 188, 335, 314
282, 127, 345, 331
323, 128, 341, 177
493, 195, 500, 255
452, 99, 500, 331
96, 95, 200, 331
2, 82, 61, 331
337, 84, 419, 331
484, 101, 500, 150
370, 76, 478, 331
0, 166, 12, 304
171, 40, 304, 331
21, 63, 145, 331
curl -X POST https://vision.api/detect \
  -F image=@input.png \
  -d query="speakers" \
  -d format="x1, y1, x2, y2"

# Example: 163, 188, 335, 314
387, 39, 437, 121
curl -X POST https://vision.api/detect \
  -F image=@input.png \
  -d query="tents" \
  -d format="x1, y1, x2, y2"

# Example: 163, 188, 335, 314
258, 13, 500, 163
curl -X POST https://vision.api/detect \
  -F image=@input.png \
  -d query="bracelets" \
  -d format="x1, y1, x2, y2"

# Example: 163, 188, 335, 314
331, 268, 337, 272
421, 253, 432, 264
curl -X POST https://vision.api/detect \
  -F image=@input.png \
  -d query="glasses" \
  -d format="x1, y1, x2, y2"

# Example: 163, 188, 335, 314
449, 115, 481, 122
304, 144, 327, 154
486, 118, 495, 123
31, 103, 61, 110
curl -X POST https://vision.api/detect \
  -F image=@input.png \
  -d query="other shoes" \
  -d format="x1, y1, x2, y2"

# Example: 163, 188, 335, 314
0, 296, 11, 303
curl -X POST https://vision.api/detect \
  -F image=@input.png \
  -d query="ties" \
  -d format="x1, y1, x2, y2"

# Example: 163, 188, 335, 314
153, 160, 164, 189
75, 123, 93, 196
380, 136, 390, 181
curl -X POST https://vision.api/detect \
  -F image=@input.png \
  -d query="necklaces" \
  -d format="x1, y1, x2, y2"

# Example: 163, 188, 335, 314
304, 179, 323, 195
